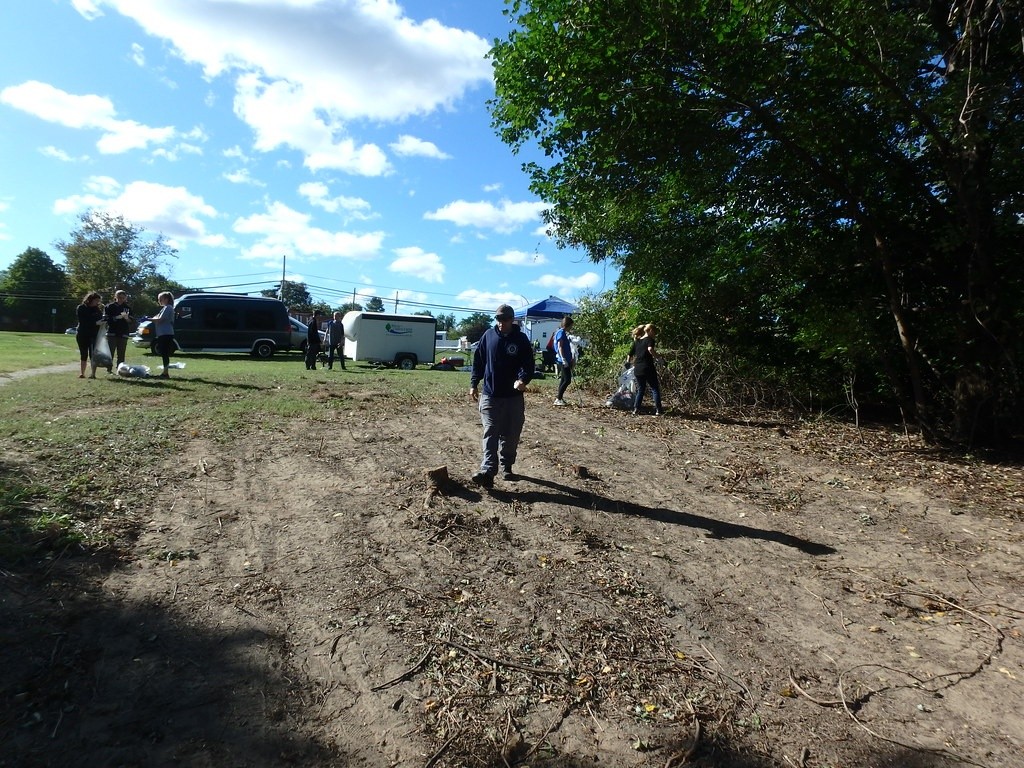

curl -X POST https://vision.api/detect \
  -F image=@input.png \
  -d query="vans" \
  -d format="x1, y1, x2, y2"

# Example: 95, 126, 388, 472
132, 295, 292, 361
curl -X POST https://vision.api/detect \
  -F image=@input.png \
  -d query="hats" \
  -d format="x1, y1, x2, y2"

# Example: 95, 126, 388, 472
494, 304, 515, 318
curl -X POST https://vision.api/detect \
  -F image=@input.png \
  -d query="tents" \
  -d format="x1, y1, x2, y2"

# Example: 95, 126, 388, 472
513, 295, 580, 334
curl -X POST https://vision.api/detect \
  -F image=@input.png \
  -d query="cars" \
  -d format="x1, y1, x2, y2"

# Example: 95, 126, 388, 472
456, 341, 480, 352
288, 316, 330, 355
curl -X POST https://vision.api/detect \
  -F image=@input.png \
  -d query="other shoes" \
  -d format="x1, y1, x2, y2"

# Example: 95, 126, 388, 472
500, 466, 513, 480
89, 374, 96, 379
633, 408, 640, 414
472, 472, 493, 488
79, 375, 85, 378
656, 409, 664, 415
554, 399, 566, 405
158, 374, 169, 379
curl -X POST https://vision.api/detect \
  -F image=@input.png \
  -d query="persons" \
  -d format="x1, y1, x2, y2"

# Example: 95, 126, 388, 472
322, 311, 348, 370
546, 316, 575, 406
469, 304, 536, 491
75, 292, 108, 379
626, 323, 668, 416
105, 289, 134, 374
138, 291, 175, 379
305, 310, 322, 370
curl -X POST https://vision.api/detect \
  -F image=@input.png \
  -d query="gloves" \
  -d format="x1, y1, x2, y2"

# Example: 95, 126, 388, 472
121, 312, 129, 320
96, 319, 107, 325
625, 362, 631, 370
659, 358, 667, 367
118, 315, 123, 319
139, 316, 148, 322
